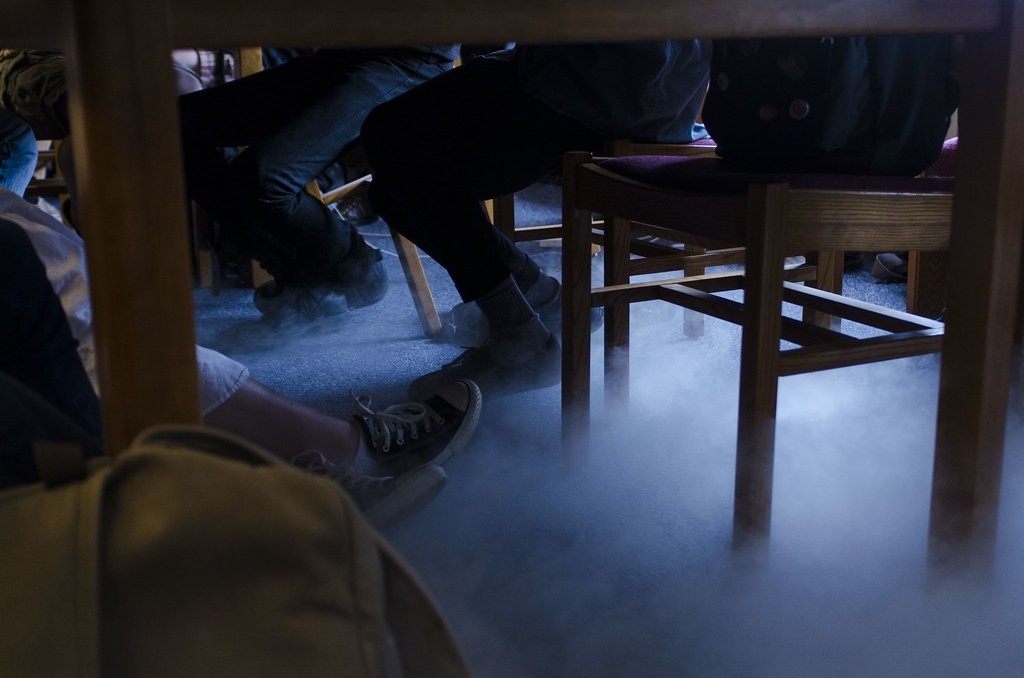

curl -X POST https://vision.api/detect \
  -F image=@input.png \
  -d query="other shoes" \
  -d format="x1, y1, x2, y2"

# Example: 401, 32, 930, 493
349, 379, 481, 502
342, 465, 446, 527
408, 337, 565, 403
253, 246, 389, 316
448, 277, 603, 343
228, 284, 346, 348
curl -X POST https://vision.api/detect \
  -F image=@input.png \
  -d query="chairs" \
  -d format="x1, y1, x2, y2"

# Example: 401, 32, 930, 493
304, 56, 954, 545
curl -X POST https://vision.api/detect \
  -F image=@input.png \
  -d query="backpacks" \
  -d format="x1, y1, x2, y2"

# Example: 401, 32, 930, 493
0, 424, 468, 678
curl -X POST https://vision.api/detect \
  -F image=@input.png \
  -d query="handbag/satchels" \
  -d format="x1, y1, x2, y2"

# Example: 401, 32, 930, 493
701, 36, 955, 174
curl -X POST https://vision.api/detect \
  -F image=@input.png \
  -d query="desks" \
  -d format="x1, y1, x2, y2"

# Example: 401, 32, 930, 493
0, 0, 1024, 678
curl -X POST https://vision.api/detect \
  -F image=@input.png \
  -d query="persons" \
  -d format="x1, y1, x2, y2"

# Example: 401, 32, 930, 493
0, 188, 481, 523
0, 46, 310, 206
356, 38, 713, 413
176, 44, 461, 318
